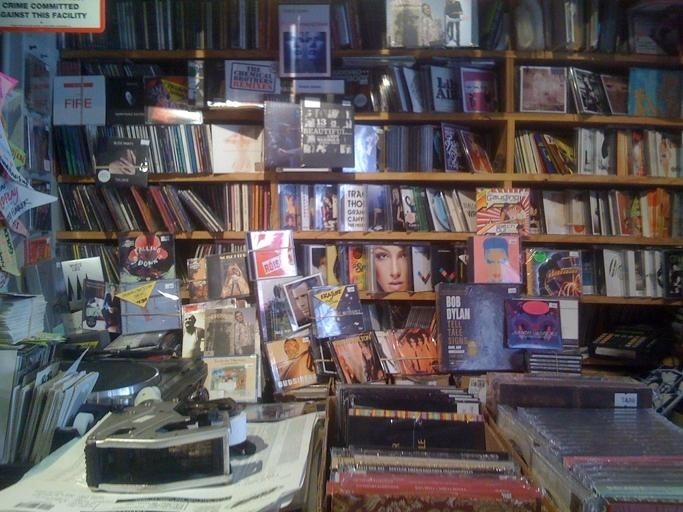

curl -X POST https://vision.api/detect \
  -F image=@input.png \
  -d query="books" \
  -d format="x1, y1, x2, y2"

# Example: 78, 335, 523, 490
326, 384, 542, 512
52, 2, 683, 405
0, 257, 104, 467
497, 405, 683, 512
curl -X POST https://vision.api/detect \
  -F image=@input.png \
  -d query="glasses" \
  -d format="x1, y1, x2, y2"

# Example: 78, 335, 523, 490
487, 259, 503, 264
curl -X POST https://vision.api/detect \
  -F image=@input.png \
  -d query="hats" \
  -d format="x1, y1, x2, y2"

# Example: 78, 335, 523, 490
483, 238, 506, 248
